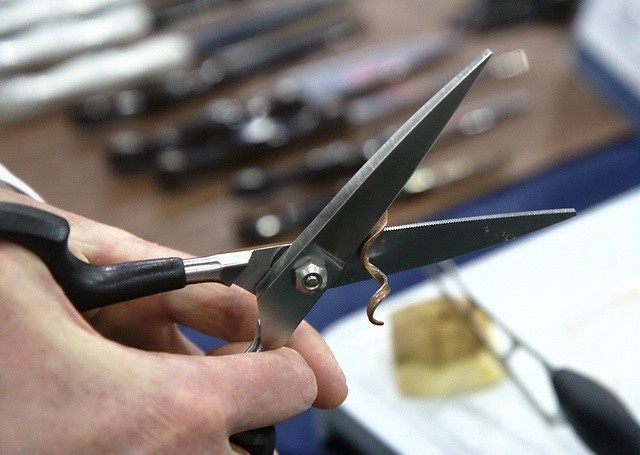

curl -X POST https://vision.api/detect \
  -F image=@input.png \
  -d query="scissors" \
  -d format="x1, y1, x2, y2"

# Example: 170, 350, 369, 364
0, 49, 576, 455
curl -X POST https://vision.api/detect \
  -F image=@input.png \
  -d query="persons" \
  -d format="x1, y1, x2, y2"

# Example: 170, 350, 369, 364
1, 163, 348, 454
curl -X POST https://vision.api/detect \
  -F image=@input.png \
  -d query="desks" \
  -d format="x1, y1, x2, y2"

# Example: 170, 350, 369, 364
308, 191, 639, 454
